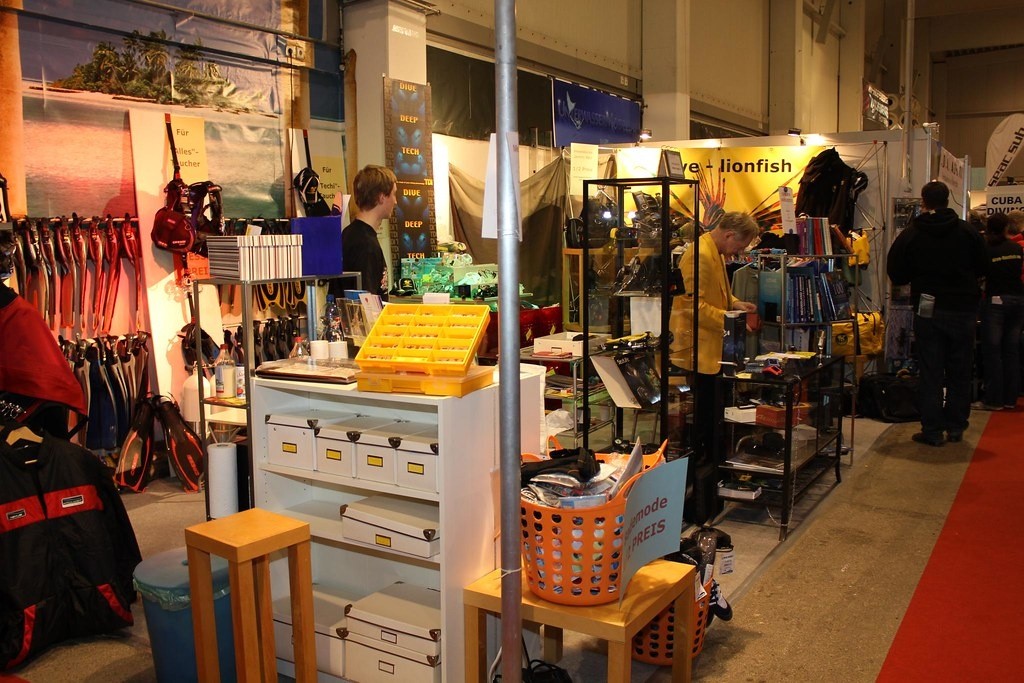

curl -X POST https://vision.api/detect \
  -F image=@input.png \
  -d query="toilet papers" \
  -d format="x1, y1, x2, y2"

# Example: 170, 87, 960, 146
310, 340, 328, 359
206, 442, 239, 519
328, 341, 349, 359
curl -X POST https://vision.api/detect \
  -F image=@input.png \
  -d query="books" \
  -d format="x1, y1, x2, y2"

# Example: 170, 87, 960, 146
759, 269, 850, 321
793, 215, 833, 255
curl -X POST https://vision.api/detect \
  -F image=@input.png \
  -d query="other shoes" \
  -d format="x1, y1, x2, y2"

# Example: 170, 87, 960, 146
911, 432, 945, 447
1004, 401, 1016, 409
947, 428, 963, 441
971, 400, 1004, 411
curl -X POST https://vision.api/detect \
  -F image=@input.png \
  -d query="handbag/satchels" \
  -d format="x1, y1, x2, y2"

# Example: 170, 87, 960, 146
817, 285, 886, 356
857, 369, 926, 424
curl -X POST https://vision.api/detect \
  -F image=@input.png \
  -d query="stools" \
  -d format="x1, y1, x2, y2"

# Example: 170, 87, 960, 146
185, 508, 317, 683
198, 408, 247, 493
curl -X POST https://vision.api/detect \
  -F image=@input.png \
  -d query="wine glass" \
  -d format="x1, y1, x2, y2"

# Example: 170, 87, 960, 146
320, 294, 343, 341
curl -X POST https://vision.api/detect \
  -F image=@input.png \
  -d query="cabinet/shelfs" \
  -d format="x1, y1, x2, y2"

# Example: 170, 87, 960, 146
563, 248, 654, 333
756, 255, 860, 465
718, 354, 847, 540
519, 346, 616, 449
193, 271, 362, 521
582, 178, 700, 526
249, 361, 548, 683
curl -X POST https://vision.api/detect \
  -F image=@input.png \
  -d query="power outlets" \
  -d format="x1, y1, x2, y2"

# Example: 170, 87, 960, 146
285, 45, 297, 58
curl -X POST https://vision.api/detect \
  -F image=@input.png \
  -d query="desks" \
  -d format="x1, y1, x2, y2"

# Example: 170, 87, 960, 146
462, 559, 696, 683
846, 352, 884, 392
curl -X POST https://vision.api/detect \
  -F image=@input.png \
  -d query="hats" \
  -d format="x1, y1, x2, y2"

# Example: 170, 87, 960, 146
921, 182, 949, 211
389, 277, 417, 297
1007, 210, 1024, 223
986, 212, 1009, 235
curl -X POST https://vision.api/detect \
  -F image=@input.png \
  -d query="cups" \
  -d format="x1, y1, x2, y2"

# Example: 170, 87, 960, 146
345, 290, 368, 300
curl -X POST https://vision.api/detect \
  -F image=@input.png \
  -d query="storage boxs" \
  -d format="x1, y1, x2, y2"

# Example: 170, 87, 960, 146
340, 496, 440, 558
353, 302, 495, 397
756, 401, 811, 428
205, 235, 303, 281
263, 408, 440, 491
271, 581, 442, 682
725, 405, 756, 423
533, 332, 613, 355
773, 424, 816, 439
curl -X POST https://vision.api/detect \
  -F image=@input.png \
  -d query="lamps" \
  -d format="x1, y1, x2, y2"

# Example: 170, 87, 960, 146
787, 128, 805, 146
635, 129, 652, 146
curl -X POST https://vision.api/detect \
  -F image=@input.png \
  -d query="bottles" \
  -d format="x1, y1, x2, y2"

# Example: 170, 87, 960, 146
234, 363, 246, 399
215, 344, 235, 397
289, 337, 309, 360
400, 258, 443, 277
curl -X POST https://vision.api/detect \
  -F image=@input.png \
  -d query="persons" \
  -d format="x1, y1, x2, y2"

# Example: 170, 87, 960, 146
669, 211, 758, 471
341, 163, 398, 302
887, 181, 992, 448
967, 206, 1024, 409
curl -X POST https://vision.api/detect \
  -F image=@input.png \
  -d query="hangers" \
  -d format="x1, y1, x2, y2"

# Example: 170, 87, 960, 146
728, 248, 840, 278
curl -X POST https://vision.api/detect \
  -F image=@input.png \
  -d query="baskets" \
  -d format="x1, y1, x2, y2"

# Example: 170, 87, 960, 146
617, 559, 712, 666
504, 432, 670, 608
